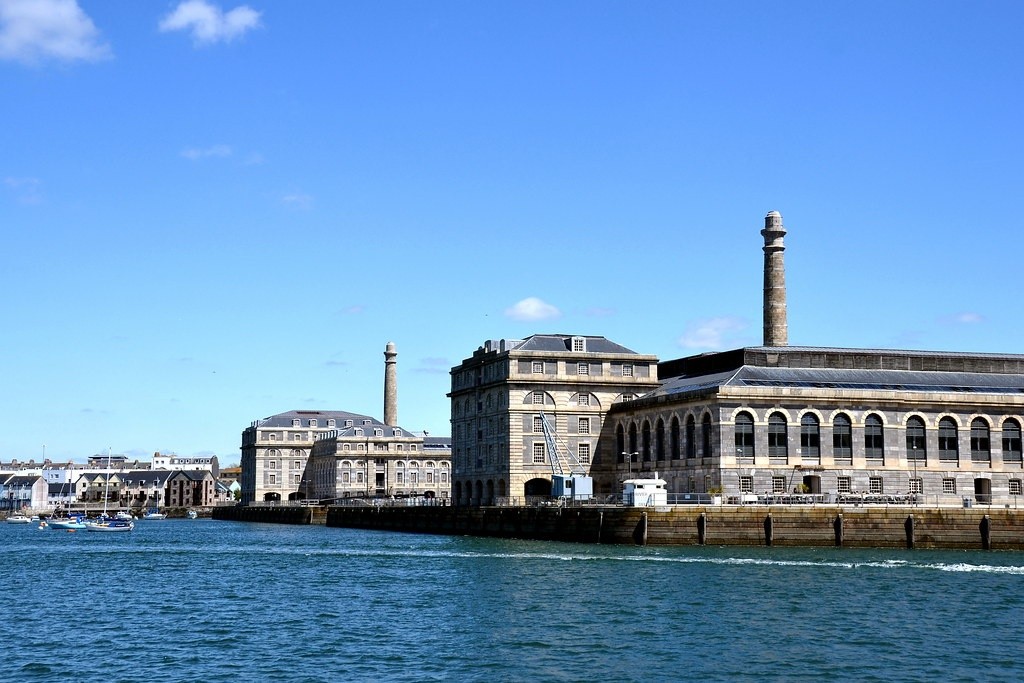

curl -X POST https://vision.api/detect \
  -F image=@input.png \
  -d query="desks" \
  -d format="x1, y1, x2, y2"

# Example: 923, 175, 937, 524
742, 493, 916, 504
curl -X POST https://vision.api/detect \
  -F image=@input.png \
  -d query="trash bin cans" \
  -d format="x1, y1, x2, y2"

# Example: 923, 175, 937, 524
963, 499, 972, 508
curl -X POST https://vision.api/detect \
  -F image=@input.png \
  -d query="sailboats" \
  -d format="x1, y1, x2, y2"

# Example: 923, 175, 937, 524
142, 476, 168, 520
44, 445, 135, 532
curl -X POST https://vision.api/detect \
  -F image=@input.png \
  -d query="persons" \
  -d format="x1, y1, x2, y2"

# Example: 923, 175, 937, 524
793, 488, 802, 504
389, 494, 395, 506
839, 489, 917, 504
53, 511, 58, 520
782, 491, 788, 504
763, 491, 770, 504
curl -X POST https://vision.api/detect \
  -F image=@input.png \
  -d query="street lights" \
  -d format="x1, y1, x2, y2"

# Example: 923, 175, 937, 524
303, 479, 312, 505
622, 451, 639, 479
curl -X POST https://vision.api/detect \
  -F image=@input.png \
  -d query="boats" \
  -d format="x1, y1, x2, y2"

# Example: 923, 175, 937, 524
6, 515, 32, 525
30, 515, 40, 522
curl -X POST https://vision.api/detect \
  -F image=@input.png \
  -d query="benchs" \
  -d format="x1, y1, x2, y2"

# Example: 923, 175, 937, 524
744, 495, 759, 504
